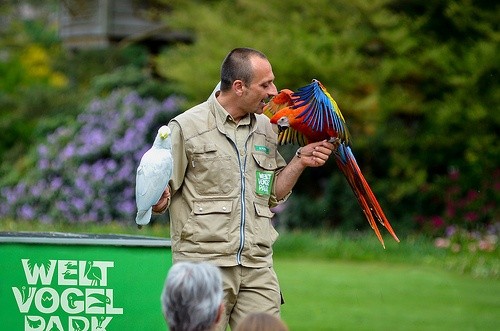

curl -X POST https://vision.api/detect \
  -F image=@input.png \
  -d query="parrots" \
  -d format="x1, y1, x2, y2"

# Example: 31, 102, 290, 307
134, 125, 174, 225
263, 79, 401, 250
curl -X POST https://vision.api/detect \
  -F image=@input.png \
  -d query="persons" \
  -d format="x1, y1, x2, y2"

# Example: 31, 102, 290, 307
159, 259, 225, 331
151, 48, 335, 330
